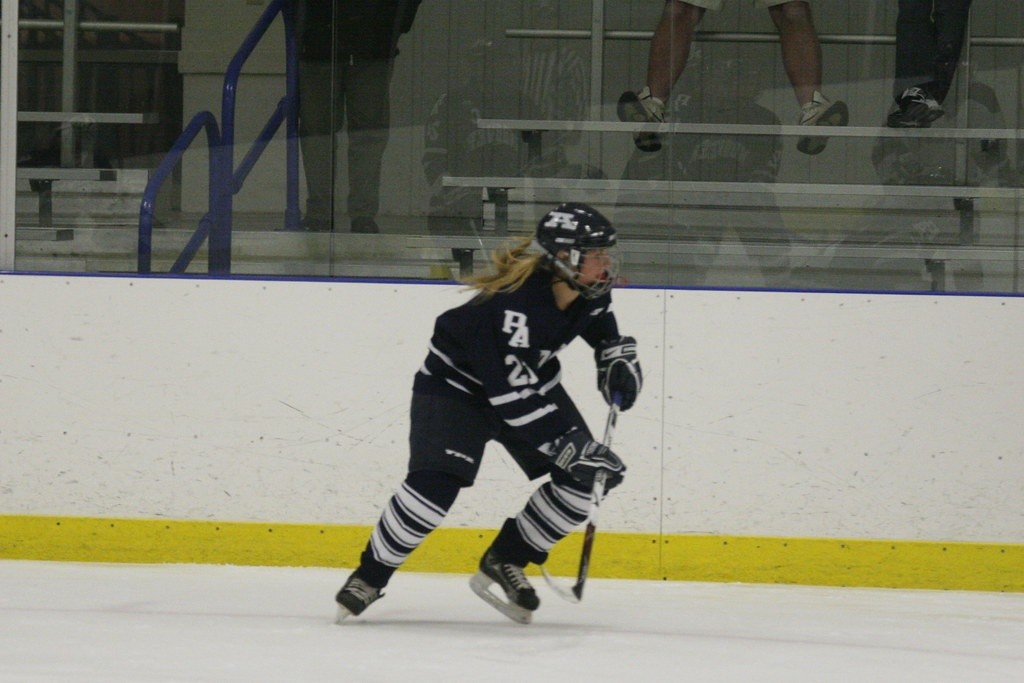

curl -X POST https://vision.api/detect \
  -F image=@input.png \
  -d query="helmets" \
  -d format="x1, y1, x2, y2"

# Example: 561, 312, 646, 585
531, 201, 620, 300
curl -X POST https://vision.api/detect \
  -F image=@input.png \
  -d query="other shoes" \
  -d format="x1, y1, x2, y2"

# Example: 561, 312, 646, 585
300, 216, 331, 232
350, 216, 379, 234
897, 87, 944, 122
887, 109, 931, 128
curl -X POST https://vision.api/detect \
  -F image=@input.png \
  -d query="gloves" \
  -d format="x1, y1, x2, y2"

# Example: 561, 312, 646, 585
595, 336, 643, 411
548, 429, 626, 491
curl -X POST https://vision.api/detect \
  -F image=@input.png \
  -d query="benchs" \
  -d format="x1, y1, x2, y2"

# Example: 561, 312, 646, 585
16, 21, 179, 227
405, 27, 1024, 295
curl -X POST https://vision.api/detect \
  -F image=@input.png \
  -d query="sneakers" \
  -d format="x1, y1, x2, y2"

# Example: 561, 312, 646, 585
617, 85, 666, 152
335, 541, 397, 624
468, 517, 539, 624
797, 90, 848, 155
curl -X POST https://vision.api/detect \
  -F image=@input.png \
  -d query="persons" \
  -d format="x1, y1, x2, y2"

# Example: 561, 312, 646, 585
421, 37, 610, 245
614, 40, 793, 289
886, 0, 972, 128
334, 202, 643, 625
619, 0, 849, 152
818, 55, 1020, 290
278, 0, 423, 236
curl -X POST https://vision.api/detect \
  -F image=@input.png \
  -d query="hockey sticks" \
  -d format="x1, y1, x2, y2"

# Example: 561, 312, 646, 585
537, 388, 625, 602
